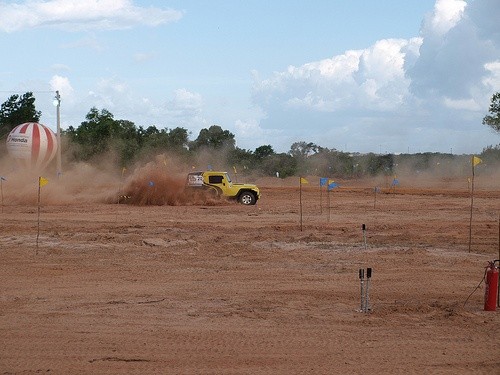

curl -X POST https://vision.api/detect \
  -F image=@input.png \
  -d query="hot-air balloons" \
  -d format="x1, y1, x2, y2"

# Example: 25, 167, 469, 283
6, 121, 59, 193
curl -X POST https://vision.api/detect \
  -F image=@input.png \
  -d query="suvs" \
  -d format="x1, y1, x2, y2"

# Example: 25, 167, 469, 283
186, 172, 261, 206
